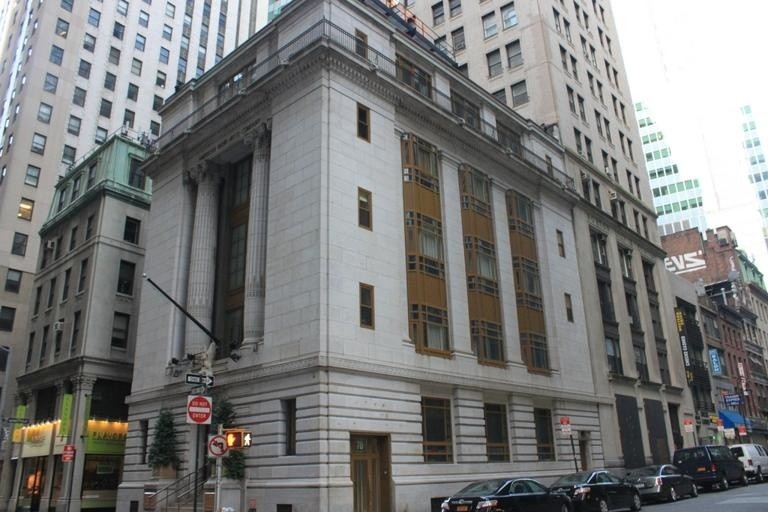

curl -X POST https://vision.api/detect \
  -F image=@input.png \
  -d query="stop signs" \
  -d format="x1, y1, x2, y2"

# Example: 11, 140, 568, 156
186, 393, 213, 426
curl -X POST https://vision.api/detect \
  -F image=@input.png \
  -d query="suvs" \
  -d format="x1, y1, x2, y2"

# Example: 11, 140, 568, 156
671, 444, 749, 491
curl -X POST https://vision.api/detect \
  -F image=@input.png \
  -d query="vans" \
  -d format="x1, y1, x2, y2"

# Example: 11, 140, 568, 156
728, 443, 768, 484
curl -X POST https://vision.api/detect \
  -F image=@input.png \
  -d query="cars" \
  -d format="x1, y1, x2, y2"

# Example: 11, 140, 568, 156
624, 462, 700, 501
547, 466, 641, 512
441, 478, 576, 512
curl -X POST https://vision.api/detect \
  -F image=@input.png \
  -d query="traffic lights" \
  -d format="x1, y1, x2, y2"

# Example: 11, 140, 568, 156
223, 429, 252, 448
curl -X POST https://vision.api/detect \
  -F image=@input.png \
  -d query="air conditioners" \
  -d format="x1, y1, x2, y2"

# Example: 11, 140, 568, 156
54, 322, 64, 332
47, 240, 55, 249
